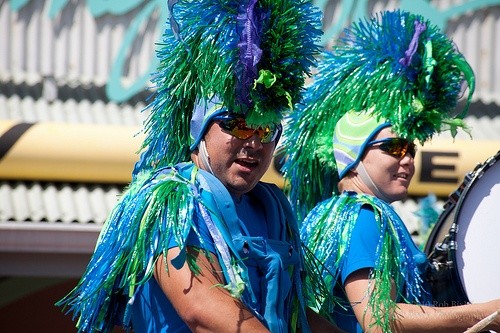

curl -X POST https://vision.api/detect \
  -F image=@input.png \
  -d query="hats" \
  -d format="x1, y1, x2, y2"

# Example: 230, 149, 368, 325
188, 87, 283, 150
333, 101, 394, 179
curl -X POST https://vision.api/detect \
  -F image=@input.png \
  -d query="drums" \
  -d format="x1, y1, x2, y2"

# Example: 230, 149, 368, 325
421, 151, 500, 333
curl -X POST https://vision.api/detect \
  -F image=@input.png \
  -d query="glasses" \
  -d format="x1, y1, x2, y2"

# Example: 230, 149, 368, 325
213, 115, 281, 144
366, 137, 417, 160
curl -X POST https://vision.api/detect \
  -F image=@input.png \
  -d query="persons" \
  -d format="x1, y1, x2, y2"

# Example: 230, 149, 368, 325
85, 79, 312, 333
297, 93, 500, 333
